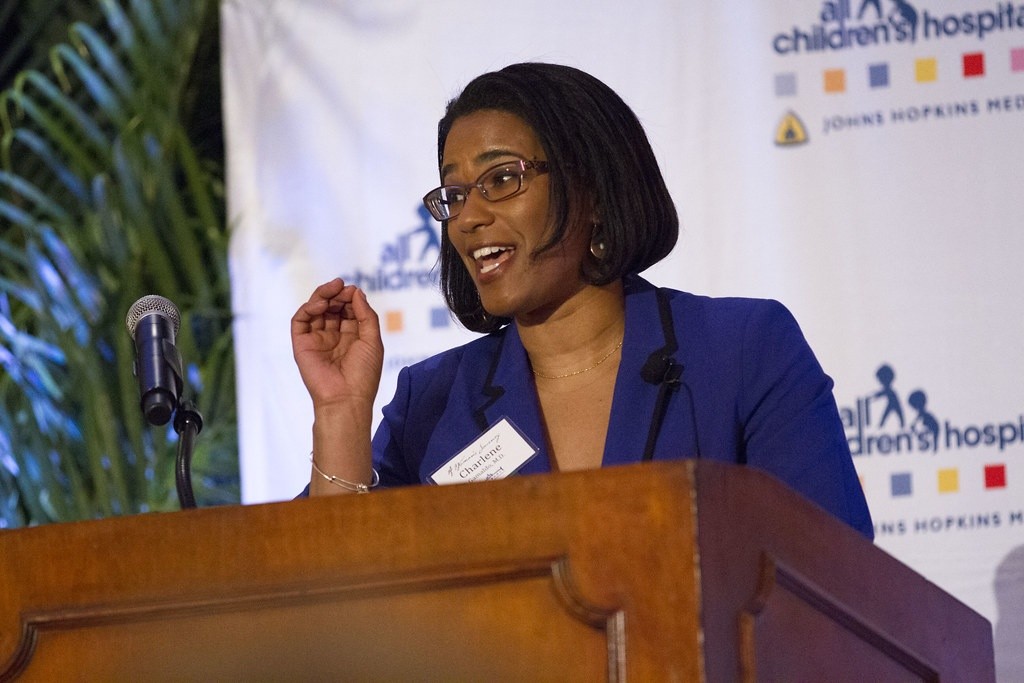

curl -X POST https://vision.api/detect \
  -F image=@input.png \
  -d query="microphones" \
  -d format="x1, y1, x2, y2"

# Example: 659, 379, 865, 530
127, 295, 180, 427
641, 354, 671, 385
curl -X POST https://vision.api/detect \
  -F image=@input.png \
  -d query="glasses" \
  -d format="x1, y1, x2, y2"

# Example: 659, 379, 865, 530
423, 160, 551, 222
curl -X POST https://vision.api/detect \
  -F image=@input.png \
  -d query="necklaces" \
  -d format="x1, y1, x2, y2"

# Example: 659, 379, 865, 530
532, 341, 622, 379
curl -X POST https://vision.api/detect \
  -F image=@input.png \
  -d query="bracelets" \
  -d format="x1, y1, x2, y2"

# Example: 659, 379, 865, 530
309, 451, 379, 494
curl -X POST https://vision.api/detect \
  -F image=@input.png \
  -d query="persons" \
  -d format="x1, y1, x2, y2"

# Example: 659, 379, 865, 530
291, 62, 875, 543
